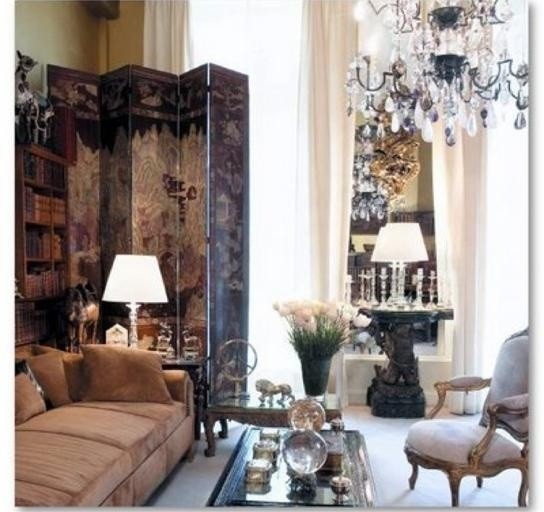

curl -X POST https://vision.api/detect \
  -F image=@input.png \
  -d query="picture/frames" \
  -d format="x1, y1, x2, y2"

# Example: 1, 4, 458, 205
104, 323, 130, 347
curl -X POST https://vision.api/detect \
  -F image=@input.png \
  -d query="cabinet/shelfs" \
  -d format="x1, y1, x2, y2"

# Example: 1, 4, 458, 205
15, 146, 72, 353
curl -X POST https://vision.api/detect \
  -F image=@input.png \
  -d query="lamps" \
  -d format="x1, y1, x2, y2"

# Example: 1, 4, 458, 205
354, 112, 407, 221
96, 253, 169, 348
384, 219, 430, 306
370, 225, 423, 311
345, 0, 529, 149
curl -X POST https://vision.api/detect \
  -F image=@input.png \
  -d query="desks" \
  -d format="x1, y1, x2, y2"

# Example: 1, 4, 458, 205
200, 392, 344, 457
354, 300, 456, 417
161, 354, 229, 443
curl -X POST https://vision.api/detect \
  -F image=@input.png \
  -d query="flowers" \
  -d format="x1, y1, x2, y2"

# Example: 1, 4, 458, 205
270, 297, 373, 360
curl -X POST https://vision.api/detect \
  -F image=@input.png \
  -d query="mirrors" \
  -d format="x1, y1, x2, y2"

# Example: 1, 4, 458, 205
346, 107, 444, 356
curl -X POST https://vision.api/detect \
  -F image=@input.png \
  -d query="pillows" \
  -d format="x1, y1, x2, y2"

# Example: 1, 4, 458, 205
15, 342, 176, 426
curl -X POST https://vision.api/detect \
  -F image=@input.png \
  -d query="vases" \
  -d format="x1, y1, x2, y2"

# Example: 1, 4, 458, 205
298, 357, 334, 396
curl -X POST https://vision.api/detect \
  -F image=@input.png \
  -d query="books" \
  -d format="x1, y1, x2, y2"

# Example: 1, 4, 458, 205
14, 150, 67, 346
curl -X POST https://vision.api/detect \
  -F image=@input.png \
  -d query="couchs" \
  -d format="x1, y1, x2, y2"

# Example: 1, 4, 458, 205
14, 340, 195, 510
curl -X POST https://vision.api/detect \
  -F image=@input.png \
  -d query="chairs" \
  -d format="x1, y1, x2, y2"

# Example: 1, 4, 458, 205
399, 327, 528, 509
